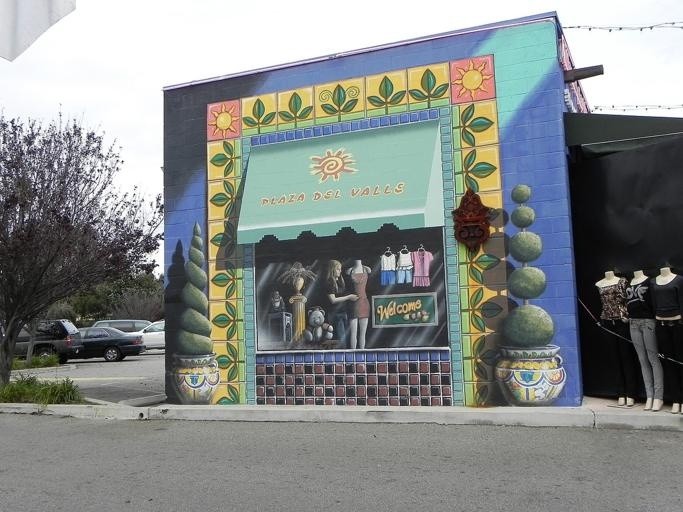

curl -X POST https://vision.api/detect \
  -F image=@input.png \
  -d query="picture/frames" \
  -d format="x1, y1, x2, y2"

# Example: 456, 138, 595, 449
371, 292, 439, 328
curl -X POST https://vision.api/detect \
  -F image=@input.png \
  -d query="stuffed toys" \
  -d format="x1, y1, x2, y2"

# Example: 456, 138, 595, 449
303, 307, 333, 342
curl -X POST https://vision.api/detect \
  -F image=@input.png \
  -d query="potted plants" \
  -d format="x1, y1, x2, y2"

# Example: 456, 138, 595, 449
169, 218, 219, 405
495, 184, 568, 407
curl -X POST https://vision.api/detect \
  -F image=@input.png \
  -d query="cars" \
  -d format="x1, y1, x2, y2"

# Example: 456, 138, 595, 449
0, 318, 165, 365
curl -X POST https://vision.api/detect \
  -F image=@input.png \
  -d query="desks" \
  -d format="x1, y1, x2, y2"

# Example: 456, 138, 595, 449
268, 312, 293, 350
257, 340, 345, 349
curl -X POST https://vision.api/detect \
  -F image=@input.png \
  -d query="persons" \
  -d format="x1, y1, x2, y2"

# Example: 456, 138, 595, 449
325, 260, 359, 341
625, 270, 665, 411
346, 259, 371, 349
595, 270, 636, 406
649, 267, 683, 414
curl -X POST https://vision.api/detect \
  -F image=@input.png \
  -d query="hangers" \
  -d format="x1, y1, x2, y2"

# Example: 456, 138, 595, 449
397, 245, 412, 254
380, 247, 397, 259
416, 244, 427, 257
